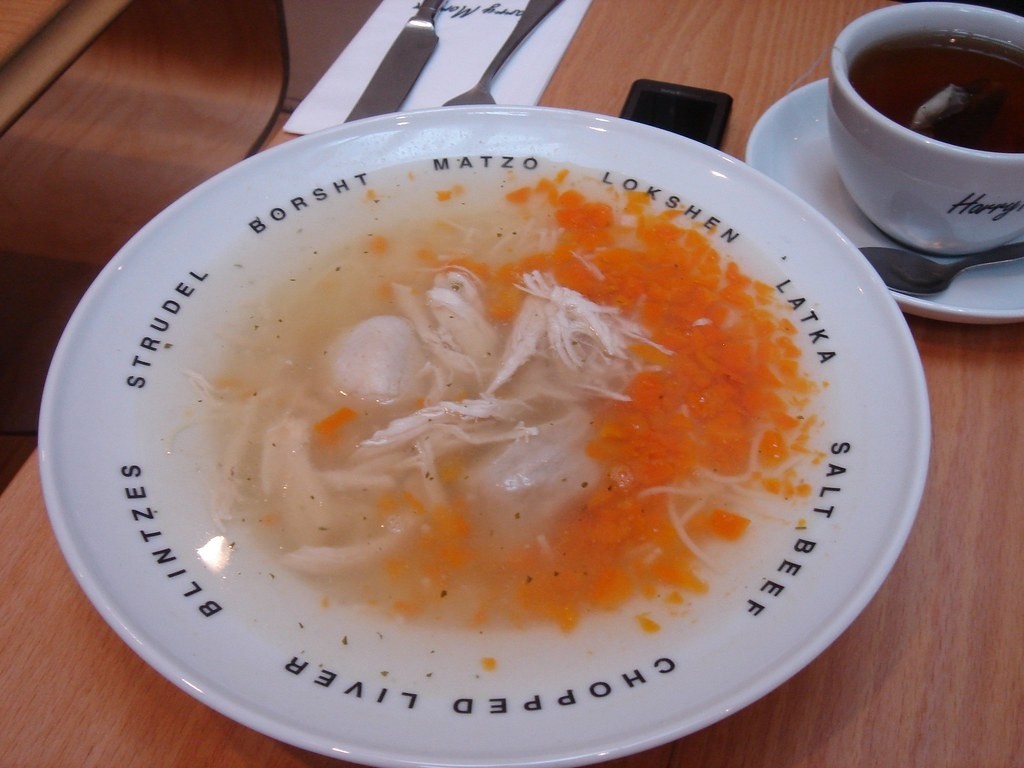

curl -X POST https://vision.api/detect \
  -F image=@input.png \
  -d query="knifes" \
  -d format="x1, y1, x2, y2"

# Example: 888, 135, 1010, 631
343, 0, 444, 123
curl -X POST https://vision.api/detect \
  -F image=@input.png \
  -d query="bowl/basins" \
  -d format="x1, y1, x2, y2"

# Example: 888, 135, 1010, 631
828, 2, 1024, 257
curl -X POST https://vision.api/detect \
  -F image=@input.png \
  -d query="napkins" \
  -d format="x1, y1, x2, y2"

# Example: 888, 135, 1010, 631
280, 0, 591, 137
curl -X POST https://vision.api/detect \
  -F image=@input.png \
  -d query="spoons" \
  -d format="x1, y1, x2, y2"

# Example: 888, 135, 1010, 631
857, 234, 1024, 296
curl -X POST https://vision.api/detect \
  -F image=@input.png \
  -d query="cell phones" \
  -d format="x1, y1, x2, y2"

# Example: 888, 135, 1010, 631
618, 79, 733, 151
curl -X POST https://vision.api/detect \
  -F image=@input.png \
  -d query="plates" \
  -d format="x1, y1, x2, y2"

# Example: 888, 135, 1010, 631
36, 103, 932, 768
745, 75, 1024, 325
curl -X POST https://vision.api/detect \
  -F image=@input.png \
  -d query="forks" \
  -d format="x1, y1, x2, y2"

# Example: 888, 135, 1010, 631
442, 0, 565, 107
